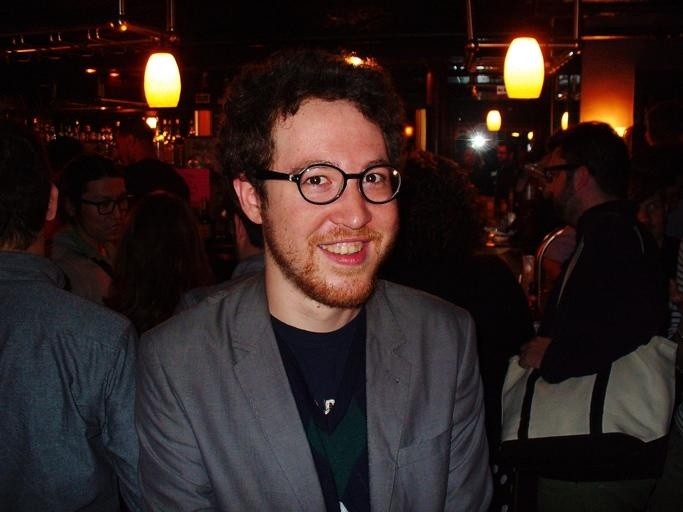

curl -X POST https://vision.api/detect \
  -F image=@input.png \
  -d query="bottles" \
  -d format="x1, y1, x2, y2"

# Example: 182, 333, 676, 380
31, 117, 183, 154
518, 255, 537, 293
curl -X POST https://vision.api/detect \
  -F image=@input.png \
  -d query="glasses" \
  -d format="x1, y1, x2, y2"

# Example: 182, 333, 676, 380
258, 162, 405, 208
542, 163, 579, 182
80, 193, 135, 216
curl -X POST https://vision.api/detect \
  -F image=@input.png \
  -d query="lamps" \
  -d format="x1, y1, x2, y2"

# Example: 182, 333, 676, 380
144, 53, 181, 108
503, 38, 544, 98
486, 110, 501, 131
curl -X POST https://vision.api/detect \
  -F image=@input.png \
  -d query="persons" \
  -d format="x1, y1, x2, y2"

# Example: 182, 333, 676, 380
382, 103, 683, 512
50, 120, 263, 317
136, 61, 493, 512
1, 119, 142, 512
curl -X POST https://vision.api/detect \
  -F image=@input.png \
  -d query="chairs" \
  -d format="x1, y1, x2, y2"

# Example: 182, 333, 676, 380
529, 226, 575, 323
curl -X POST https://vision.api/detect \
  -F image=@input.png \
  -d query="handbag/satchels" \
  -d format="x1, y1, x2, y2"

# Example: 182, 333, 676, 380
493, 328, 680, 484
